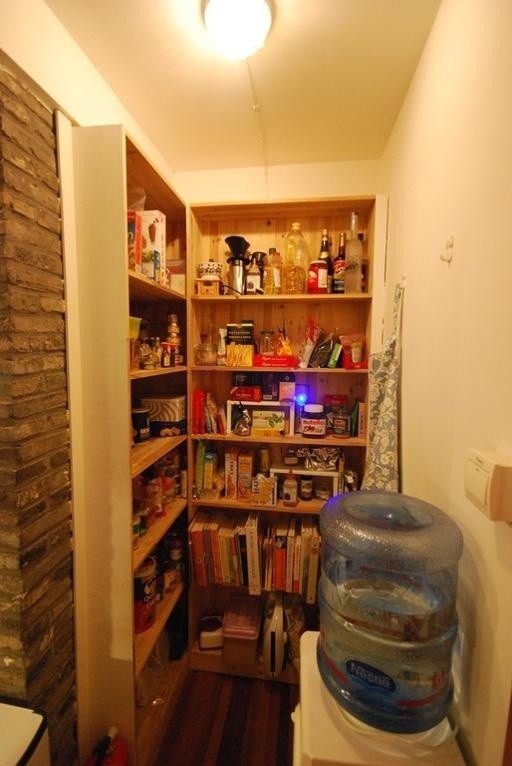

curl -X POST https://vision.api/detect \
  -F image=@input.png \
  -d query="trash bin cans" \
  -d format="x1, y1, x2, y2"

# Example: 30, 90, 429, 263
0, 697, 51, 766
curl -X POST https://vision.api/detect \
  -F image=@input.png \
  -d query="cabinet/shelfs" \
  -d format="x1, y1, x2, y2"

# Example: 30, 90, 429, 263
52, 112, 188, 765
186, 193, 388, 683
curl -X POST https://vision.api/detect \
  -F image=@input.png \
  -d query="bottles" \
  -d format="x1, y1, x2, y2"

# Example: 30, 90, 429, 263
244, 212, 367, 294
282, 467, 314, 507
195, 344, 218, 367
169, 533, 183, 561
260, 328, 275, 357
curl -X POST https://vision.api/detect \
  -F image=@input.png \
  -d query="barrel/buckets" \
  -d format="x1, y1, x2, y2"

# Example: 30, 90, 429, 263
315, 490, 464, 734
281, 222, 308, 293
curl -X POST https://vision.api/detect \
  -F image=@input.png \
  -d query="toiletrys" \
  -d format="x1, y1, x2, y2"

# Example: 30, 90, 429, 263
282, 466, 299, 507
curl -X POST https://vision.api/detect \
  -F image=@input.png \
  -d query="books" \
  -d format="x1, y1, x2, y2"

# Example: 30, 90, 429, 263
186, 505, 324, 604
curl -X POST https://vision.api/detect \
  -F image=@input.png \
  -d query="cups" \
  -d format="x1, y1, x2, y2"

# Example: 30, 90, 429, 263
197, 263, 222, 280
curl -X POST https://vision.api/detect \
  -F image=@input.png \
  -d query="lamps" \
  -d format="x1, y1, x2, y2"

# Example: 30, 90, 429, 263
199, 0, 276, 60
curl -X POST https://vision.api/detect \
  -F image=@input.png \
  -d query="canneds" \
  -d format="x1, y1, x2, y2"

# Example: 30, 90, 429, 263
135, 556, 158, 633
306, 260, 329, 294
132, 407, 151, 443
158, 561, 176, 594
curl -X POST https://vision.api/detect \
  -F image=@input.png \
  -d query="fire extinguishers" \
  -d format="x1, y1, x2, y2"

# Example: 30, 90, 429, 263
87, 726, 129, 766
